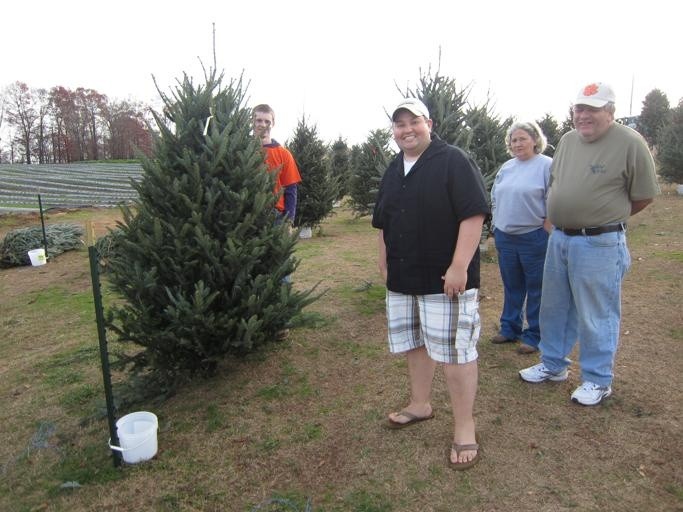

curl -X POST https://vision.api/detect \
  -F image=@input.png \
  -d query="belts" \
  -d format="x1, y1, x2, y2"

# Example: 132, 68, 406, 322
556, 223, 627, 236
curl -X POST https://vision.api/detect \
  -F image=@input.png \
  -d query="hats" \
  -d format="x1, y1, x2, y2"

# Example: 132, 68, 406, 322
573, 80, 615, 107
392, 97, 430, 118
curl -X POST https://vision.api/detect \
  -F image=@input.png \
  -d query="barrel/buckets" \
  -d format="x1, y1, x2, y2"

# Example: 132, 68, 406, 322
28, 249, 49, 267
299, 227, 312, 239
108, 412, 159, 463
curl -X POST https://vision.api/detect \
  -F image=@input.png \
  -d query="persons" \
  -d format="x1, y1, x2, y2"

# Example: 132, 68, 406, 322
517, 80, 661, 407
487, 120, 554, 354
369, 97, 492, 472
247, 104, 302, 342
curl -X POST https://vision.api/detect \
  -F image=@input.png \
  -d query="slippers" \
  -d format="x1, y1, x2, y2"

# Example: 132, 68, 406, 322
384, 411, 435, 429
448, 442, 479, 470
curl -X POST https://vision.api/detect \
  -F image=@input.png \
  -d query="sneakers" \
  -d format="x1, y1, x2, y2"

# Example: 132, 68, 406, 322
518, 344, 540, 354
489, 335, 520, 343
518, 362, 568, 382
571, 381, 611, 405
